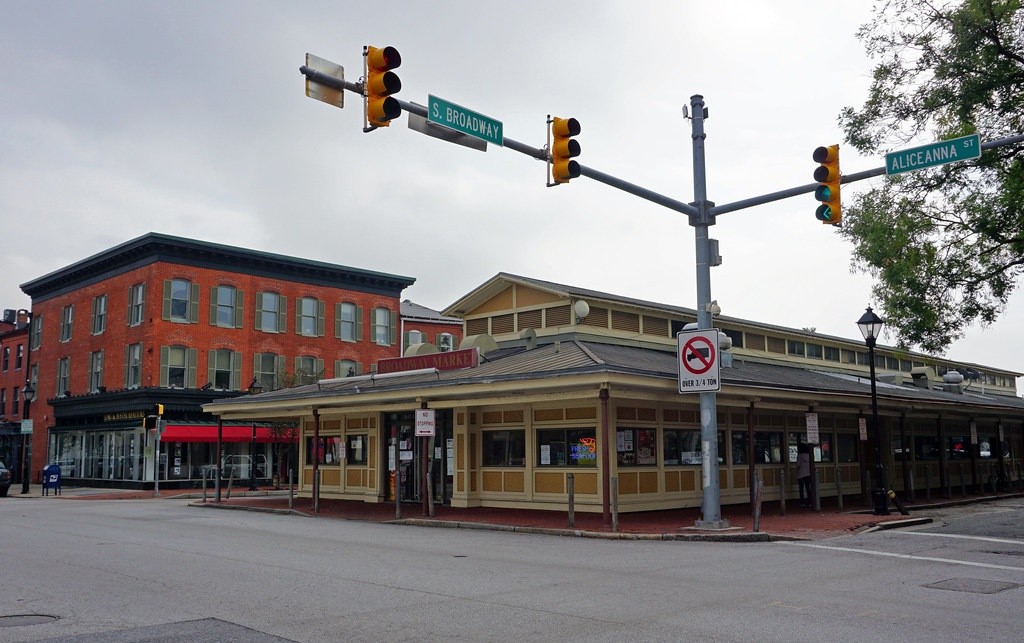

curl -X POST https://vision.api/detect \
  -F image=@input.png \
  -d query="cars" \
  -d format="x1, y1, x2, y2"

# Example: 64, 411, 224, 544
0, 461, 12, 497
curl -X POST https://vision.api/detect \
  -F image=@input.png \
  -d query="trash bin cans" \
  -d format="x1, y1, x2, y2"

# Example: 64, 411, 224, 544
42, 464, 61, 488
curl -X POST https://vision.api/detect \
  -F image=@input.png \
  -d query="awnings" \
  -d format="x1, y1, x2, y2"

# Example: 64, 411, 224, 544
161, 426, 299, 442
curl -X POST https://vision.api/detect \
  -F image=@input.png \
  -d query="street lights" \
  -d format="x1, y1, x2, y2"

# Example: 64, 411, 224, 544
855, 303, 891, 515
21, 379, 34, 494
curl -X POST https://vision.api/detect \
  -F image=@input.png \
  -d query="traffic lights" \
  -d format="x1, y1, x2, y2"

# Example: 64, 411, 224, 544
144, 416, 157, 427
367, 45, 402, 128
812, 143, 843, 228
551, 117, 581, 183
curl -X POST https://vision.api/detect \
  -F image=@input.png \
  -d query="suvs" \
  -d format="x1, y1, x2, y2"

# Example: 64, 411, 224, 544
198, 451, 277, 478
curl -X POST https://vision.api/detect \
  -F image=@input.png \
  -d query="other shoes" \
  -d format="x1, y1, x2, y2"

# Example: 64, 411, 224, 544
808, 503, 812, 506
800, 504, 806, 506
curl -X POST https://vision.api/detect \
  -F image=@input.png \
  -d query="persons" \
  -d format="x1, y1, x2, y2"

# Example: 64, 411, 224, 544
869, 450, 884, 487
796, 444, 812, 506
